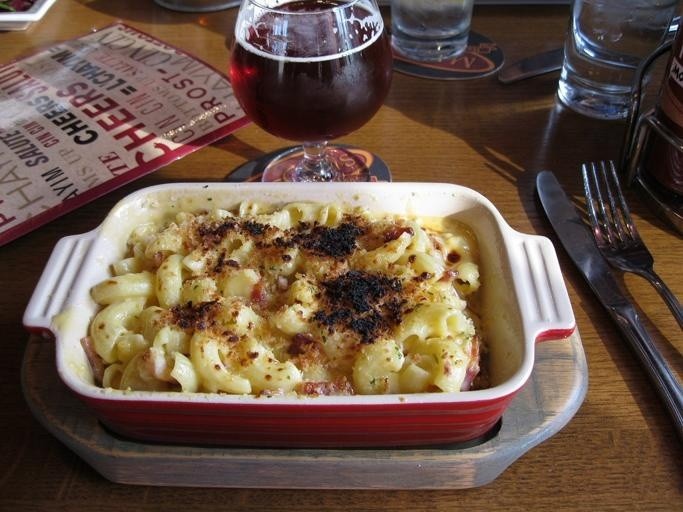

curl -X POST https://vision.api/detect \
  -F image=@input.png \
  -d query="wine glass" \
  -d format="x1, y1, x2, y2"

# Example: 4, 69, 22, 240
229, 0, 392, 185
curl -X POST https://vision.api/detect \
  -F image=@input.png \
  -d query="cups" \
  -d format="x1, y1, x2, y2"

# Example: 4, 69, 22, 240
392, 1, 473, 62
558, 1, 677, 120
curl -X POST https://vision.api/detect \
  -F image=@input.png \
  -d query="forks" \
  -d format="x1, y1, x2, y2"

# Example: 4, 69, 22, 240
582, 161, 683, 332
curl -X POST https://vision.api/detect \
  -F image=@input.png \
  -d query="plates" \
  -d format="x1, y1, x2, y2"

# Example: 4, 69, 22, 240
158, 0, 244, 11
22, 326, 588, 490
383, 27, 503, 80
0, 0, 55, 30
226, 143, 390, 183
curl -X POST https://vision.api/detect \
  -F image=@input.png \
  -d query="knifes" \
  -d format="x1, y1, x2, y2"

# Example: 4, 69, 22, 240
536, 171, 683, 445
497, 14, 682, 84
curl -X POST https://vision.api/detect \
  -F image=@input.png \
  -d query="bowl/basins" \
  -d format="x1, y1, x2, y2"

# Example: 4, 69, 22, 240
23, 183, 575, 448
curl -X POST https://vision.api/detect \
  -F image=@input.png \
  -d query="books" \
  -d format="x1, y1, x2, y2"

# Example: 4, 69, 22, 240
0, 22, 254, 246
